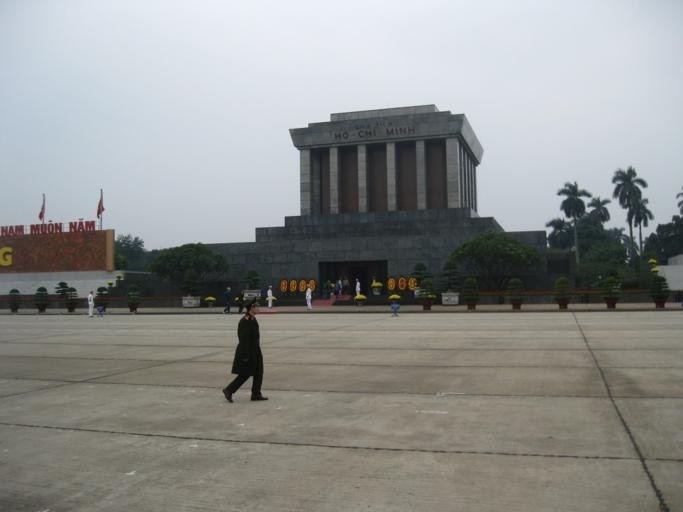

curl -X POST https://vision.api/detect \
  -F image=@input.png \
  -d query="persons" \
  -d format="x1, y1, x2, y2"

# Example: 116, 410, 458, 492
237, 289, 244, 313
223, 297, 269, 403
323, 276, 361, 299
265, 286, 273, 307
223, 285, 231, 314
305, 286, 313, 312
87, 290, 95, 318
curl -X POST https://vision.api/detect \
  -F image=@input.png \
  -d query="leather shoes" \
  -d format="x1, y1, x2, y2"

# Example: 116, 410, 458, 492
251, 396, 268, 400
223, 389, 234, 402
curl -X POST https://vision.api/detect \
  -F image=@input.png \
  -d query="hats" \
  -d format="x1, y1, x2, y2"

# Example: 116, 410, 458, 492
246, 296, 260, 307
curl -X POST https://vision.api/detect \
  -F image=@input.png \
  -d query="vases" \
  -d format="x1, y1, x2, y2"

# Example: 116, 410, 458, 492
356, 301, 363, 307
390, 301, 400, 318
208, 302, 212, 307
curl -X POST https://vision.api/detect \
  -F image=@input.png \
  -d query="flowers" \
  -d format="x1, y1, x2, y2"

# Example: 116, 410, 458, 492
204, 296, 216, 304
353, 281, 401, 301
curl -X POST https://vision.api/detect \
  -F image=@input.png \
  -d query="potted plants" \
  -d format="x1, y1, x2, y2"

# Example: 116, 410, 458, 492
147, 240, 230, 309
6, 281, 145, 313
242, 268, 262, 299
416, 275, 673, 311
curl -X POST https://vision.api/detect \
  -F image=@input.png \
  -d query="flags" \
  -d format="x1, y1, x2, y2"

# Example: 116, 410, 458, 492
39, 193, 46, 220
96, 191, 105, 219
